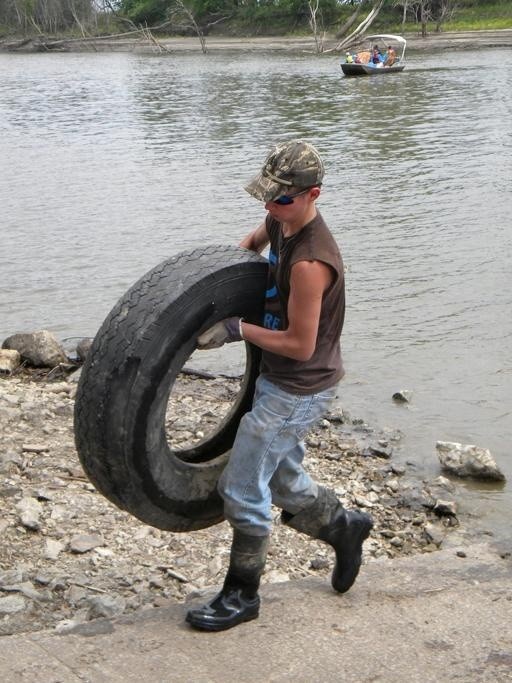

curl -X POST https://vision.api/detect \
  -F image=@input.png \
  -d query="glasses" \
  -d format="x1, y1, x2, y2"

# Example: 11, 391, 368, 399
273, 188, 310, 205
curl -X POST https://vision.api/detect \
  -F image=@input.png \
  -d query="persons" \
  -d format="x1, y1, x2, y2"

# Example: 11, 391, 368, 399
372, 45, 396, 68
344, 51, 358, 64
182, 138, 372, 633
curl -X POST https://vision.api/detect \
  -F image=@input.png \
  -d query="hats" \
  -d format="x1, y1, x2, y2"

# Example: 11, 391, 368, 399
242, 140, 324, 201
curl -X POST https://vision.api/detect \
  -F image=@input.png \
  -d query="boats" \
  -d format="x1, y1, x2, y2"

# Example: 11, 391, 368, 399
340, 34, 406, 75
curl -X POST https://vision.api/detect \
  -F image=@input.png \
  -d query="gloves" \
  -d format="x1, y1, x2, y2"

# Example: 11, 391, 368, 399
196, 316, 244, 349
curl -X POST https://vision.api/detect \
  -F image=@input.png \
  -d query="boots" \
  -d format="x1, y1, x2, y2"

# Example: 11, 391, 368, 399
281, 486, 372, 592
186, 529, 269, 631
